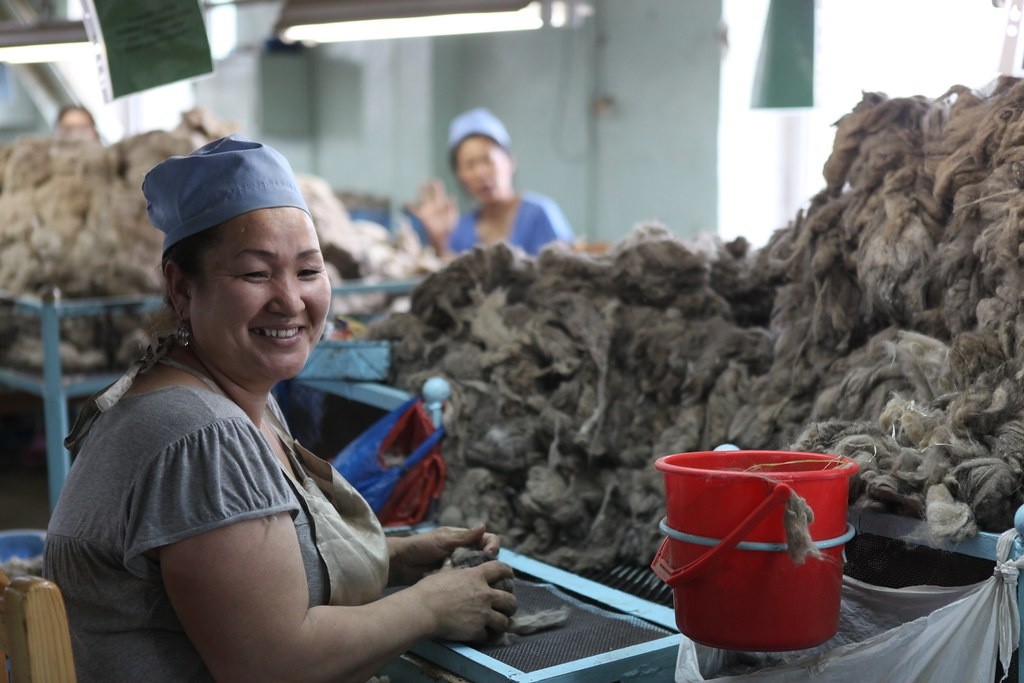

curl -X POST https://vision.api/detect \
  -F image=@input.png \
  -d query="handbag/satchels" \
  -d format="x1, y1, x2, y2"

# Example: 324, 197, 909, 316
329, 392, 450, 527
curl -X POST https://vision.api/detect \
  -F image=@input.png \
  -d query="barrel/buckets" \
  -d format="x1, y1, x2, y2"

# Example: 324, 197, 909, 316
0, 528, 47, 683
653, 450, 860, 651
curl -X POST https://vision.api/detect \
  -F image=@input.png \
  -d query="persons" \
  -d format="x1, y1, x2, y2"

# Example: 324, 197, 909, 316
405, 107, 572, 260
43, 129, 518, 683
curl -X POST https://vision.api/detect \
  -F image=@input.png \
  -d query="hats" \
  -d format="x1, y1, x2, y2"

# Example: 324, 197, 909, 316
142, 133, 312, 258
446, 109, 512, 169
